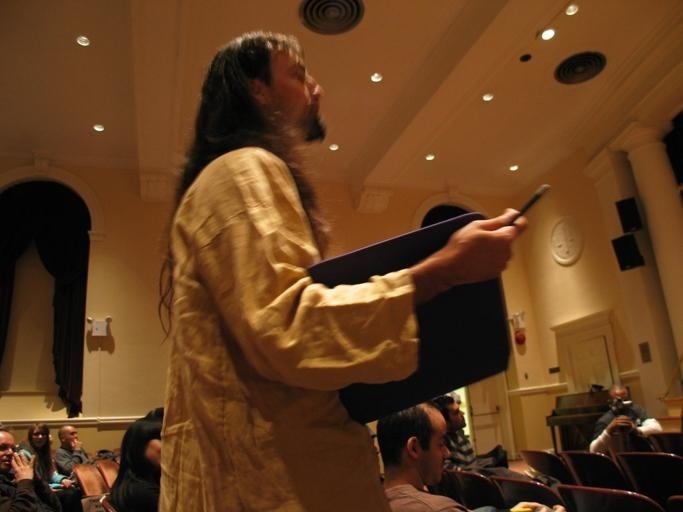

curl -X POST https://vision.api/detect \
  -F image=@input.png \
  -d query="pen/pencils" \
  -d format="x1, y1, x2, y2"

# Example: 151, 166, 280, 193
514, 183, 553, 219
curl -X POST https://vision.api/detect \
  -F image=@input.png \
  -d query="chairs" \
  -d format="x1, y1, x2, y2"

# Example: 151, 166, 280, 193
424, 422, 682, 511
70, 448, 125, 512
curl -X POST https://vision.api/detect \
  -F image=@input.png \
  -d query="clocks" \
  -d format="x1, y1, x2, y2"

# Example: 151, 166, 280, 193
548, 216, 585, 265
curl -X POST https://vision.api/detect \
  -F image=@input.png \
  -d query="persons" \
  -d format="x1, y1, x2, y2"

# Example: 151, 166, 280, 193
0, 408, 214, 512
376, 403, 569, 512
428, 393, 501, 476
152, 33, 529, 512
586, 386, 660, 467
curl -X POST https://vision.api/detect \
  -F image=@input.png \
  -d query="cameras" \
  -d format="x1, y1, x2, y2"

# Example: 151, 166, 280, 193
606, 397, 635, 428
